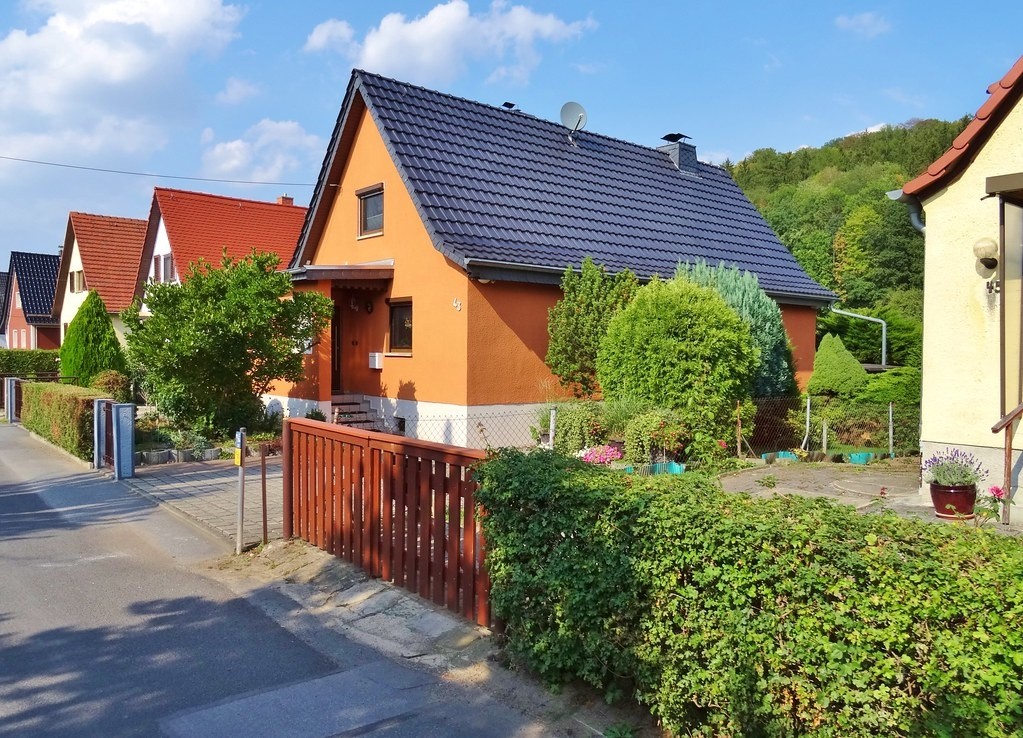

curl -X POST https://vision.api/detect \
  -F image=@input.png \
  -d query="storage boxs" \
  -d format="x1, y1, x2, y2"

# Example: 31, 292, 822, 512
368, 353, 382, 369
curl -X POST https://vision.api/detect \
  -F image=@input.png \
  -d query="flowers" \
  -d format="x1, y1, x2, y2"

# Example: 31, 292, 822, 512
919, 447, 990, 486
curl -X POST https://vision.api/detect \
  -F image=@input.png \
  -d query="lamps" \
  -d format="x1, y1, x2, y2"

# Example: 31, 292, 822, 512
973, 237, 999, 269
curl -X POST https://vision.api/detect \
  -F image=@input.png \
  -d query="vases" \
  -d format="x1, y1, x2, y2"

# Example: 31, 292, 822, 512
929, 481, 979, 519
